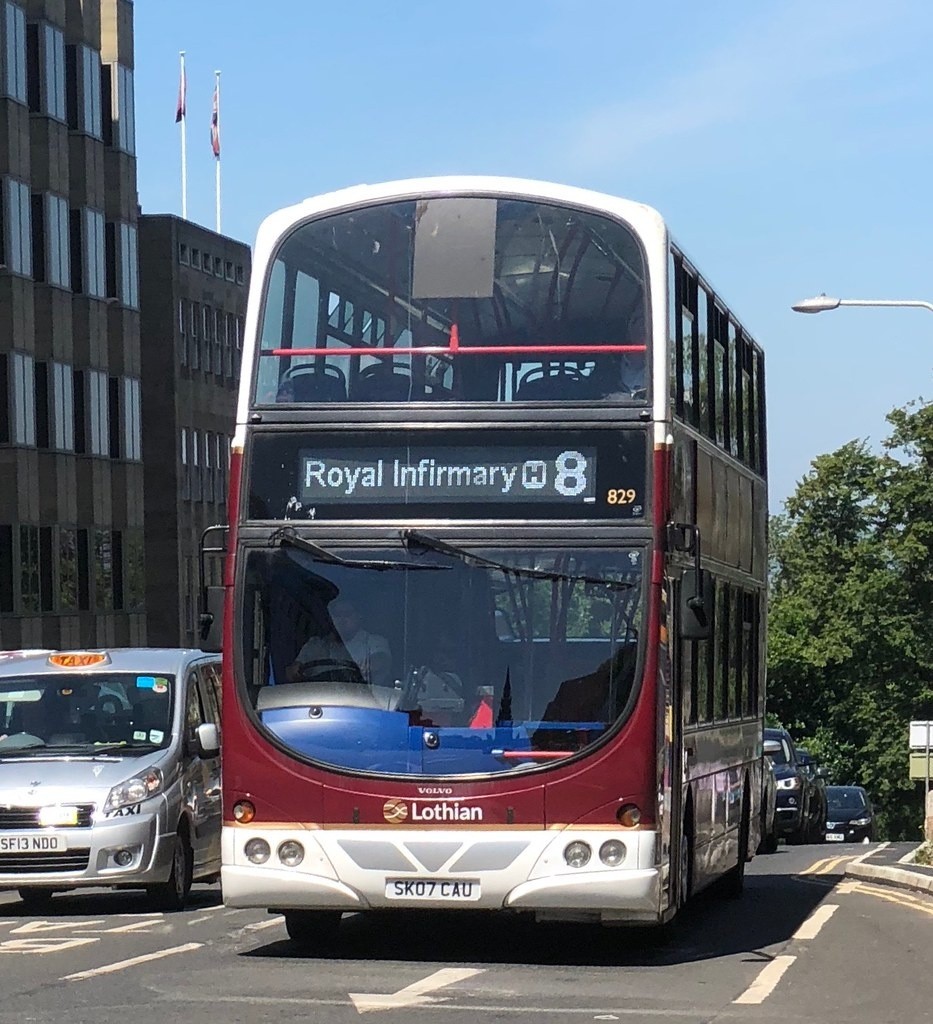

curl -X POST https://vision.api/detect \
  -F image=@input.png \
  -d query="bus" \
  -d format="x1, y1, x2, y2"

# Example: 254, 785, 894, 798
197, 175, 771, 949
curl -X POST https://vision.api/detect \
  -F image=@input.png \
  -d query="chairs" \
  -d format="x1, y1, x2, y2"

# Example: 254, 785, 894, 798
275, 363, 347, 402
348, 361, 423, 399
515, 365, 594, 398
132, 698, 168, 741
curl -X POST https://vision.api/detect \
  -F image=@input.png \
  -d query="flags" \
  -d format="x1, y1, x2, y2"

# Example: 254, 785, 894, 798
174, 58, 187, 124
208, 75, 221, 160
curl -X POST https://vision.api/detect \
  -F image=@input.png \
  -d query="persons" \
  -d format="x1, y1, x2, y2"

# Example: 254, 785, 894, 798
286, 594, 396, 686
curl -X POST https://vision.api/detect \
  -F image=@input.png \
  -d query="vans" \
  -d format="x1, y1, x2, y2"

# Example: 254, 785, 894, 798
0, 647, 225, 916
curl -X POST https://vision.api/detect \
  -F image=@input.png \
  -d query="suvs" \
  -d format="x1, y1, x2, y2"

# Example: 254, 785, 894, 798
759, 725, 827, 853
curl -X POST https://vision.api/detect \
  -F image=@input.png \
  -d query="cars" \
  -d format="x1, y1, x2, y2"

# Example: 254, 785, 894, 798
824, 784, 874, 843
